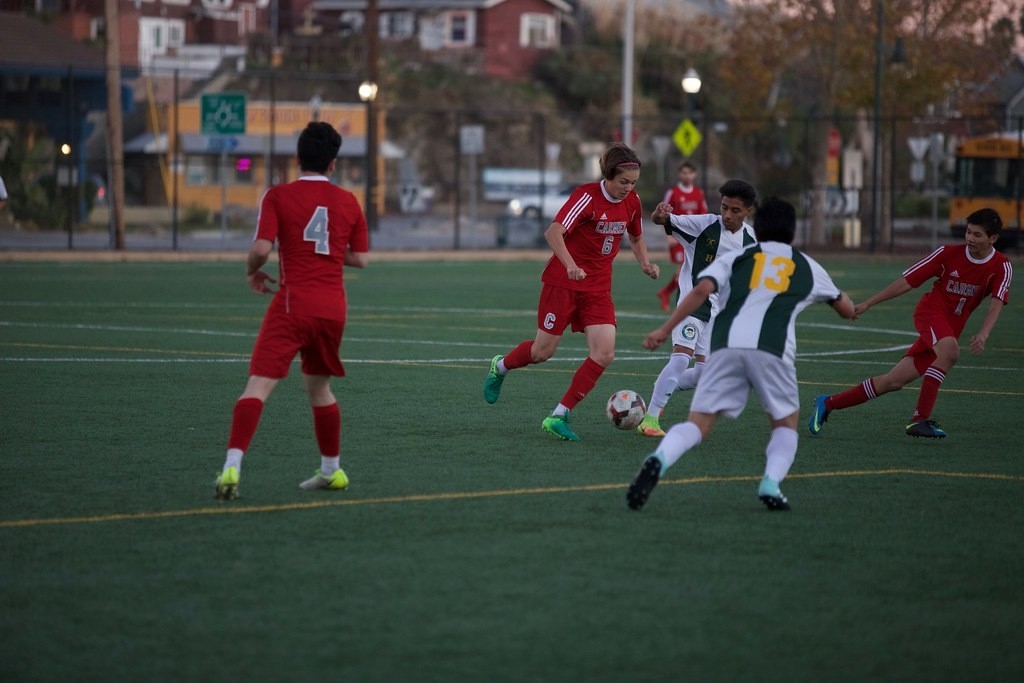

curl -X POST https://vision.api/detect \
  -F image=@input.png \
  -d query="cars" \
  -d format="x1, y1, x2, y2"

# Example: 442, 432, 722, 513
507, 181, 582, 224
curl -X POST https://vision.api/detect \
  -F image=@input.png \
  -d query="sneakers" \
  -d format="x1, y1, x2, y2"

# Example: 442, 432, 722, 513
626, 457, 662, 512
759, 478, 790, 511
482, 354, 505, 404
809, 394, 831, 434
541, 415, 580, 441
906, 418, 946, 439
658, 289, 669, 311
635, 416, 666, 438
298, 469, 350, 491
213, 469, 239, 500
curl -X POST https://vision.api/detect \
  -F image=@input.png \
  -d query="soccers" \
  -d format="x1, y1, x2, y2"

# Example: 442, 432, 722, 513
606, 389, 647, 430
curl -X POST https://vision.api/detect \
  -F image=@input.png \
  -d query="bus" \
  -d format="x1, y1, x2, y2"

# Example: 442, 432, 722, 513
482, 166, 562, 203
949, 128, 1024, 248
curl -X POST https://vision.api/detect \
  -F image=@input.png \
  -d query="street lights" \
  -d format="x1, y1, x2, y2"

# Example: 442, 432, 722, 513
678, 62, 703, 164
356, 76, 382, 234
867, 0, 910, 255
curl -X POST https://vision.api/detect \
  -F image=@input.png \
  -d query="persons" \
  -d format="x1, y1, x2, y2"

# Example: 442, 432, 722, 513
217, 121, 369, 498
627, 198, 855, 511
658, 164, 707, 309
484, 144, 660, 441
0, 177, 9, 209
808, 209, 1013, 439
638, 181, 757, 435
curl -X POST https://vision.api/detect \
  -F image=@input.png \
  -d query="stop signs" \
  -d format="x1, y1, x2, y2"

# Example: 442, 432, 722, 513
827, 127, 843, 156
